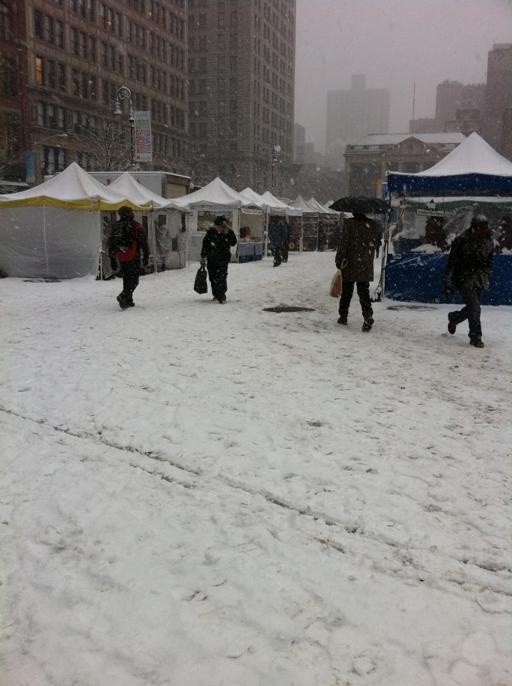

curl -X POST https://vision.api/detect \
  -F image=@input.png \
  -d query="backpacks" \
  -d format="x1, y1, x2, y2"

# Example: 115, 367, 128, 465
111, 219, 136, 248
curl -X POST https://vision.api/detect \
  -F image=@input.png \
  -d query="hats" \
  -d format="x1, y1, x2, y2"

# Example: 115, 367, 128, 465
119, 206, 134, 220
215, 215, 227, 225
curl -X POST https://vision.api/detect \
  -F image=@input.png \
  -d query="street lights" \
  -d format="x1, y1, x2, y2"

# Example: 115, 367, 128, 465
1, 133, 71, 171
270, 144, 283, 193
114, 82, 135, 170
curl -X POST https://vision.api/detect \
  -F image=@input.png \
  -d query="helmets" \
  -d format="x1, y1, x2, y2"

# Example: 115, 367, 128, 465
471, 214, 488, 230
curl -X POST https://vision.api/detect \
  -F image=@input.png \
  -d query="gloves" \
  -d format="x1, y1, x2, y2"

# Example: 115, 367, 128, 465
111, 258, 118, 271
200, 258, 206, 267
143, 251, 150, 265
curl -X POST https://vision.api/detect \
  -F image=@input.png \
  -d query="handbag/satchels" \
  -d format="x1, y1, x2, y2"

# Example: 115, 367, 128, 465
330, 269, 342, 297
194, 267, 207, 294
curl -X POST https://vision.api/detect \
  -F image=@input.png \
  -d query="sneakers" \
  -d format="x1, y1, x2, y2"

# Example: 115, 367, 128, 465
117, 291, 135, 307
470, 336, 484, 347
448, 312, 455, 334
363, 318, 374, 332
338, 316, 347, 324
213, 297, 227, 304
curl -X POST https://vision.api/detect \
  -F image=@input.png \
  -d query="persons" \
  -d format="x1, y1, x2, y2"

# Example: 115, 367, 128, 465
200, 215, 237, 304
332, 212, 379, 332
328, 195, 393, 216
442, 214, 495, 347
268, 215, 342, 267
107, 206, 150, 310
